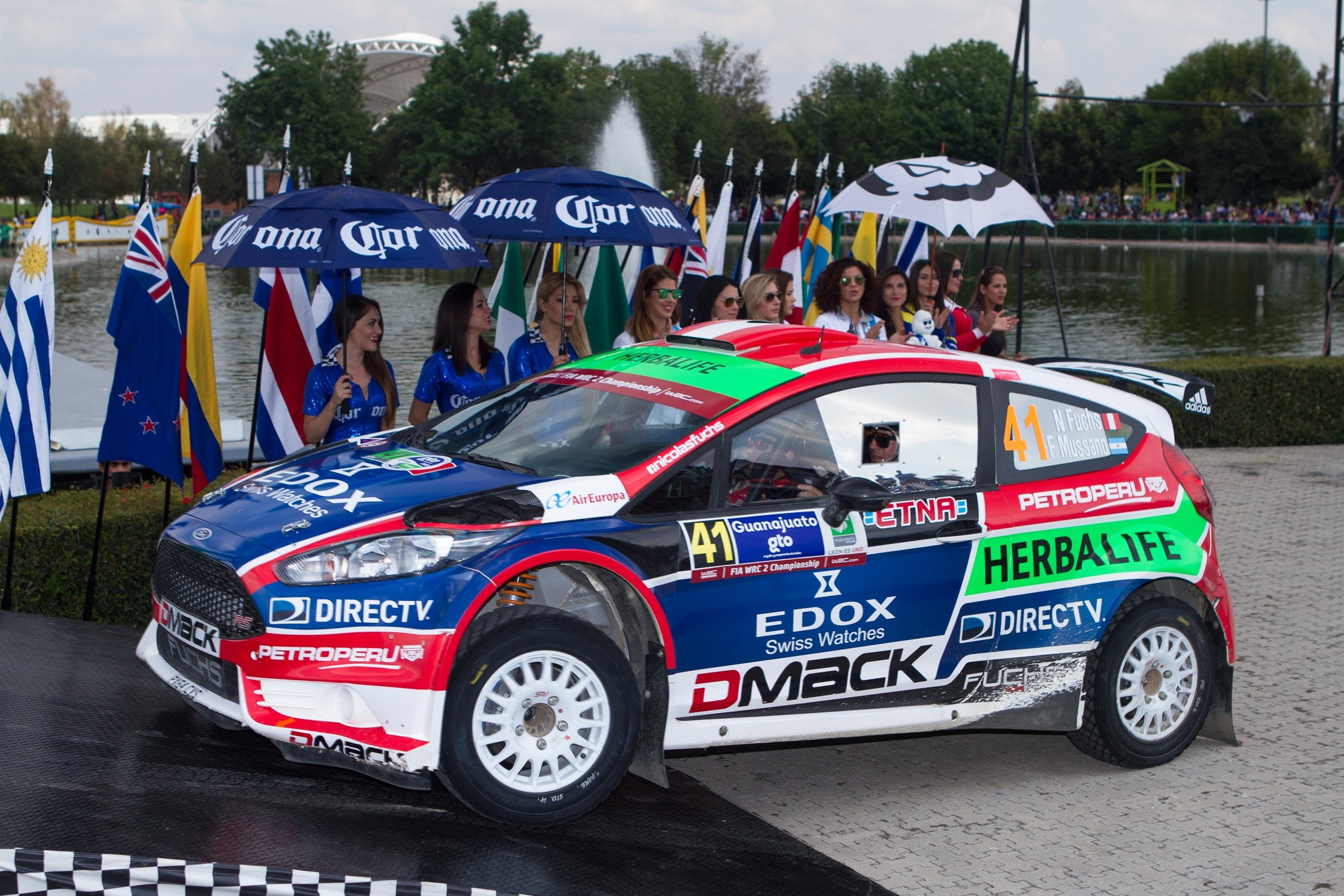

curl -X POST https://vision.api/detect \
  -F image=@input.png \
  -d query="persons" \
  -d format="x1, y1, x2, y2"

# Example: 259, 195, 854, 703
811, 256, 889, 341
932, 248, 1020, 353
909, 259, 957, 350
408, 282, 506, 426
611, 265, 684, 350
739, 268, 797, 324
794, 427, 900, 500
876, 266, 917, 344
697, 275, 748, 325
968, 265, 1034, 362
1042, 176, 1343, 225
508, 272, 593, 387
93, 200, 169, 221
301, 297, 400, 445
0, 208, 34, 258
99, 461, 132, 480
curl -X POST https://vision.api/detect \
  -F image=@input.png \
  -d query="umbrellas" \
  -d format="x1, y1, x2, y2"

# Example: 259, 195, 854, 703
820, 156, 1055, 306
188, 171, 494, 421
447, 152, 708, 355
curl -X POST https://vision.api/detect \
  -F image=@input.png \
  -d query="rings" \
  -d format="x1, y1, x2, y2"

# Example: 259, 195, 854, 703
1007, 323, 1009, 328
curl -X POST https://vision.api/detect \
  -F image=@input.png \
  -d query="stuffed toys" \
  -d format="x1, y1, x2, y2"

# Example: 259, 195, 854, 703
905, 309, 942, 348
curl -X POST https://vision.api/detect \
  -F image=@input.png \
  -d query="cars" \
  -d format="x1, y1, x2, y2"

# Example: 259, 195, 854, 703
134, 316, 1242, 827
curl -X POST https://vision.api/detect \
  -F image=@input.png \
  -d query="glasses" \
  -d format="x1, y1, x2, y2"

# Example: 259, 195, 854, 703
839, 275, 866, 286
746, 436, 774, 452
952, 269, 963, 278
646, 288, 684, 300
715, 297, 745, 308
864, 429, 896, 448
764, 292, 784, 302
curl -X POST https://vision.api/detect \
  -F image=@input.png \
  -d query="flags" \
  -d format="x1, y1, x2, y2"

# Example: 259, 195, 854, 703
663, 173, 708, 322
896, 219, 932, 277
801, 183, 836, 328
629, 244, 656, 319
851, 208, 879, 274
574, 252, 630, 354
96, 203, 187, 490
705, 182, 735, 275
526, 236, 565, 331
831, 186, 845, 262
486, 241, 530, 386
738, 196, 765, 290
166, 185, 226, 498
220, 175, 340, 462
763, 190, 809, 326
0, 196, 55, 524
312, 260, 366, 365
878, 215, 891, 267
